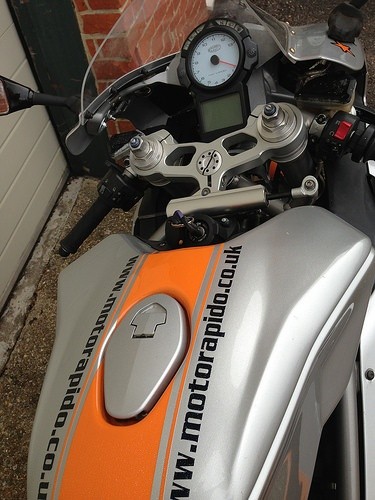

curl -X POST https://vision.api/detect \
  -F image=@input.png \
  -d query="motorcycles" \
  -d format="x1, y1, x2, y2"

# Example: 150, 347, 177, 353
0, 0, 375, 500
